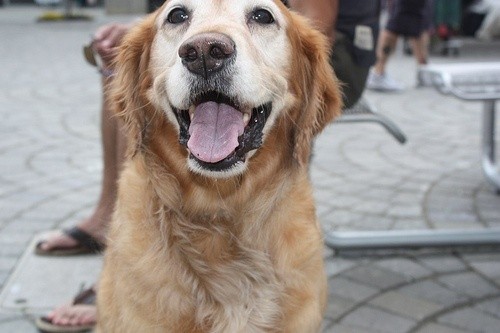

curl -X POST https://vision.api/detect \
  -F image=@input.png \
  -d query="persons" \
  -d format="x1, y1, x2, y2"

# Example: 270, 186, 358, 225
368, 0, 462, 91
35, 0, 377, 332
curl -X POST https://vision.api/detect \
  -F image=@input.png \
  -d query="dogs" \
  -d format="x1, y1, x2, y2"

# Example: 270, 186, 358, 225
88, 0, 347, 333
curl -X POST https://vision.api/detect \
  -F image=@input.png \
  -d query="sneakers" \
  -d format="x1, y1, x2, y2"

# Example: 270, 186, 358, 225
367, 71, 387, 88
419, 69, 442, 86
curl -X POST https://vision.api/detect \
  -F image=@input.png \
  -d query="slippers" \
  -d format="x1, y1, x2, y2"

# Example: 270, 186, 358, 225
36, 289, 98, 333
34, 226, 107, 256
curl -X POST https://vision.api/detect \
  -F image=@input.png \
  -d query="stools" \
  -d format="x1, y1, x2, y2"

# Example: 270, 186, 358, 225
311, 100, 408, 254
420, 36, 500, 190
